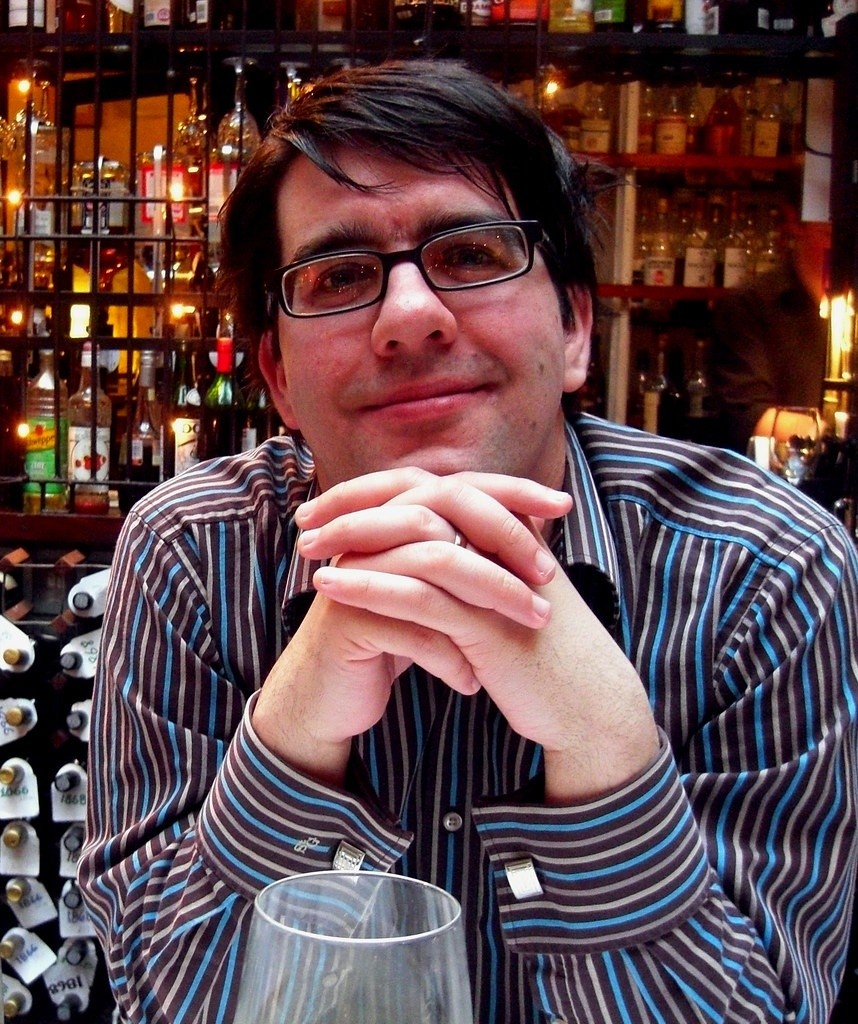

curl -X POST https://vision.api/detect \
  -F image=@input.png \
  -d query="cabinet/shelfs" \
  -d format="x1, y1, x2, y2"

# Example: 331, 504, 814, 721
566, 81, 805, 426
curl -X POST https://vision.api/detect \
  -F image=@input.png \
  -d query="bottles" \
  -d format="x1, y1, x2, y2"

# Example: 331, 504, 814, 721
24, 348, 68, 514
204, 337, 244, 459
16, 125, 238, 321
682, 199, 717, 287
555, 82, 793, 159
1, 350, 22, 503
242, 388, 289, 452
120, 350, 164, 513
164, 340, 204, 482
720, 192, 748, 286
0, 568, 104, 1024
68, 341, 112, 514
0, 0, 858, 35
643, 198, 676, 287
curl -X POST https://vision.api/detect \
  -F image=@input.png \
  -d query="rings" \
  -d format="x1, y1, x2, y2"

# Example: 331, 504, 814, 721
454, 530, 468, 548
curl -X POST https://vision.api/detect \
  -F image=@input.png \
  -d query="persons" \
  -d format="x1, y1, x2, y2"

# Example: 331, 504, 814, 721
77, 59, 858, 1024
107, 255, 156, 382
142, 245, 160, 270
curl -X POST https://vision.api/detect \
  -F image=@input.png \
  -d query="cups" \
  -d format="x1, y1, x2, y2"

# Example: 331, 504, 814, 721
231, 870, 473, 1024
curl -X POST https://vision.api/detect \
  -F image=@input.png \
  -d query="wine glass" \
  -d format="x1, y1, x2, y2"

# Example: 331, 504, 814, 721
216, 57, 259, 164
172, 77, 210, 160
